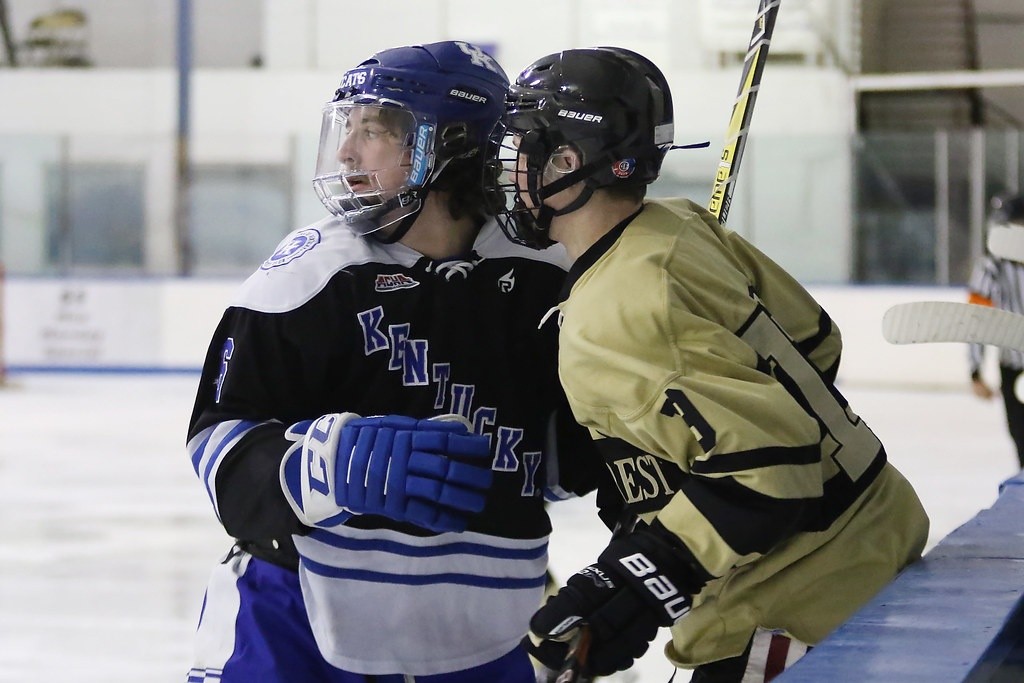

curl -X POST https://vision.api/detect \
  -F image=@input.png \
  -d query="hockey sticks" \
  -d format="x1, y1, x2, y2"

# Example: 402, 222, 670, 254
880, 299, 1024, 357
555, 507, 641, 682
705, 0, 781, 228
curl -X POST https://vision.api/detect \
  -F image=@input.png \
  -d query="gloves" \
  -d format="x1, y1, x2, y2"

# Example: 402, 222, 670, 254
280, 412, 494, 534
521, 521, 707, 678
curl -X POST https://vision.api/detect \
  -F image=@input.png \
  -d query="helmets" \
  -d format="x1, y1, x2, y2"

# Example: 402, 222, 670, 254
482, 46, 674, 251
313, 40, 509, 243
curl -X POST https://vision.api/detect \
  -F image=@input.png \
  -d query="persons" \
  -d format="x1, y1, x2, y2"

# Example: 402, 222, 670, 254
482, 47, 930, 683
184, 40, 600, 683
965, 192, 1024, 473
